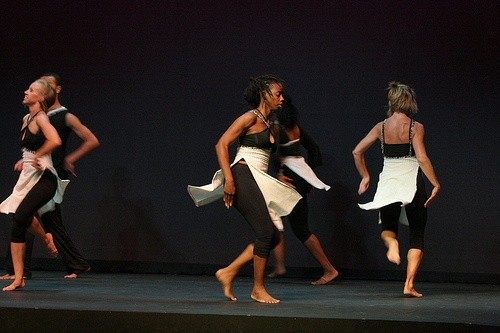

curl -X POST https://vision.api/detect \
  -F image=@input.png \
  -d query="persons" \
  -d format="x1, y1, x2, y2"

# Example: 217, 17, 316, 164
352, 81, 440, 297
187, 75, 303, 304
0, 73, 99, 280
0, 79, 70, 291
267, 90, 339, 286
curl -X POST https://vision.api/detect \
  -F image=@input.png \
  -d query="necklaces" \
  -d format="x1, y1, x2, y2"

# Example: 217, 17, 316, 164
249, 109, 270, 127
